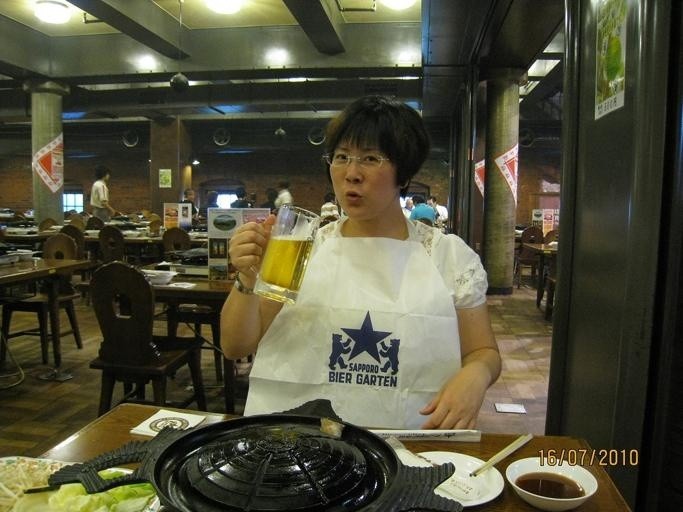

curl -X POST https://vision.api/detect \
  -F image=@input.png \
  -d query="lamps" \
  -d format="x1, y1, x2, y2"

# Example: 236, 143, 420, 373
189, 153, 200, 166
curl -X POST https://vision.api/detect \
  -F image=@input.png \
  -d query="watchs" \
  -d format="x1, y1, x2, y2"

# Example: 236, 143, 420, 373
234, 272, 256, 297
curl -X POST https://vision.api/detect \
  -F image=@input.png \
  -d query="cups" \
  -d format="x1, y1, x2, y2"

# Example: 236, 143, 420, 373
247, 203, 322, 307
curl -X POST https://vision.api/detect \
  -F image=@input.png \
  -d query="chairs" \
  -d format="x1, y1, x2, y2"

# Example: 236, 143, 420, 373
70, 218, 84, 231
31, 218, 58, 251
163, 227, 191, 265
149, 215, 161, 221
65, 213, 83, 220
0, 233, 83, 370
149, 220, 162, 236
129, 214, 139, 222
99, 225, 138, 303
60, 225, 88, 284
514, 227, 543, 289
86, 217, 104, 229
544, 230, 559, 244
142, 209, 151, 218
89, 261, 206, 417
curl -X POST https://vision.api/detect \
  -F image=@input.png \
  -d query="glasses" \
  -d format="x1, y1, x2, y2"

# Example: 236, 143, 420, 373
325, 151, 389, 170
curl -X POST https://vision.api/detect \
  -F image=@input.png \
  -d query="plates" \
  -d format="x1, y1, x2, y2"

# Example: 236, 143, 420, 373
86, 230, 100, 237
124, 231, 140, 237
1, 454, 160, 512
400, 450, 506, 508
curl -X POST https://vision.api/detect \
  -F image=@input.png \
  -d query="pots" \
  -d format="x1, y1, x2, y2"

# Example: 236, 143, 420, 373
46, 413, 463, 512
169, 247, 209, 266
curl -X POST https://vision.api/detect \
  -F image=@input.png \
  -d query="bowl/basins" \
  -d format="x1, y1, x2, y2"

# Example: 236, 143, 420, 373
141, 270, 178, 284
19, 254, 33, 261
505, 456, 600, 512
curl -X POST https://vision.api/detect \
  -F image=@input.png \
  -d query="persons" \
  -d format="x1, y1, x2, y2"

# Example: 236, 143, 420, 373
230, 186, 250, 208
262, 188, 277, 210
221, 97, 501, 431
416, 218, 432, 227
405, 199, 414, 212
409, 196, 437, 223
183, 188, 196, 219
274, 179, 293, 214
426, 198, 448, 222
91, 164, 115, 221
321, 193, 338, 217
199, 190, 220, 219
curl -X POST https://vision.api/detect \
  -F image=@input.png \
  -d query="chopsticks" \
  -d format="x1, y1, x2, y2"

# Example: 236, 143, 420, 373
469, 434, 535, 480
